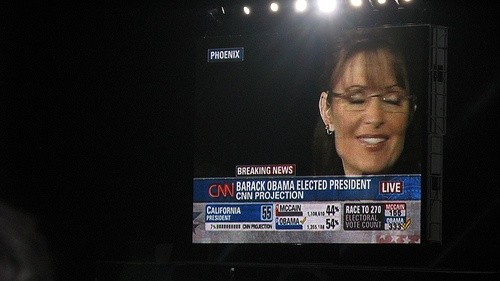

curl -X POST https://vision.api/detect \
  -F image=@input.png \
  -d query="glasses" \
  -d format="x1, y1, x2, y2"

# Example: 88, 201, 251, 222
326, 89, 417, 113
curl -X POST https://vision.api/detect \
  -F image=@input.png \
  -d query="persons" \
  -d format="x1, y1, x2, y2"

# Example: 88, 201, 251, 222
308, 32, 419, 177
0, 204, 55, 281
